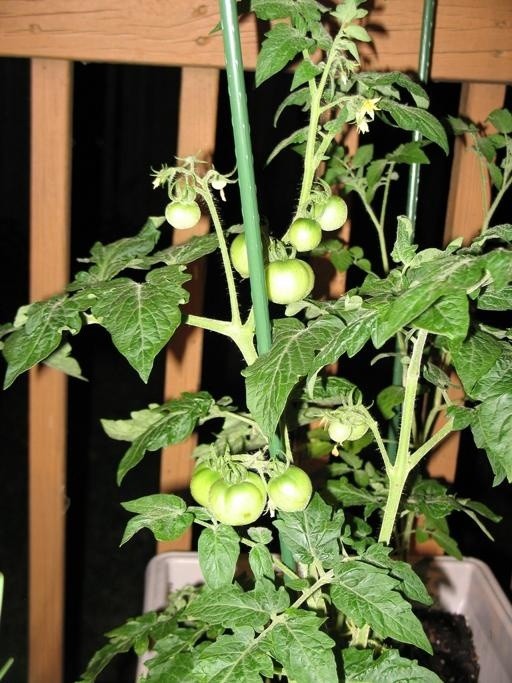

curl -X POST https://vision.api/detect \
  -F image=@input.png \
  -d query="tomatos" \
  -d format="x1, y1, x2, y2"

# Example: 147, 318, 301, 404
161, 190, 370, 525
